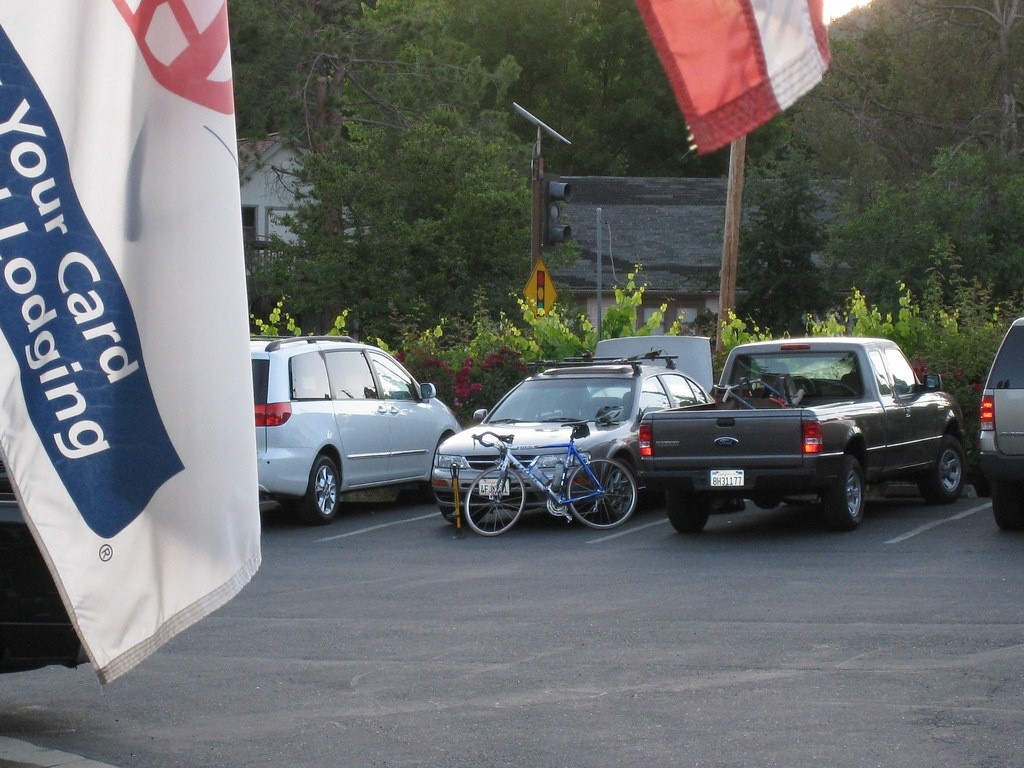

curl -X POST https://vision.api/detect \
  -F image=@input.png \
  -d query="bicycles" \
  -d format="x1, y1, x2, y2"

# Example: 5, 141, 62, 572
465, 419, 637, 538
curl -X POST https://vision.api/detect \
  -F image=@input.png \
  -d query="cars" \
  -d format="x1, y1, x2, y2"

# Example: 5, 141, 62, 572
430, 337, 716, 525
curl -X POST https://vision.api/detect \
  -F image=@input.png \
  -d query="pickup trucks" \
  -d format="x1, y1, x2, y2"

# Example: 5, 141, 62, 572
638, 338, 969, 532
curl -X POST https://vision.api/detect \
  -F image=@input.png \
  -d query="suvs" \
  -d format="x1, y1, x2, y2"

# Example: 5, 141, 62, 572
978, 318, 1024, 531
251, 336, 464, 524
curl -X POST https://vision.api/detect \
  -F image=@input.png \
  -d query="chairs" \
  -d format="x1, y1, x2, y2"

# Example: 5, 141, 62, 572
762, 363, 791, 391
841, 374, 858, 393
582, 397, 624, 422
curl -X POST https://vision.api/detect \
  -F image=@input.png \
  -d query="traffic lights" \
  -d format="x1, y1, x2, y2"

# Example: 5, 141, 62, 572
540, 177, 571, 251
